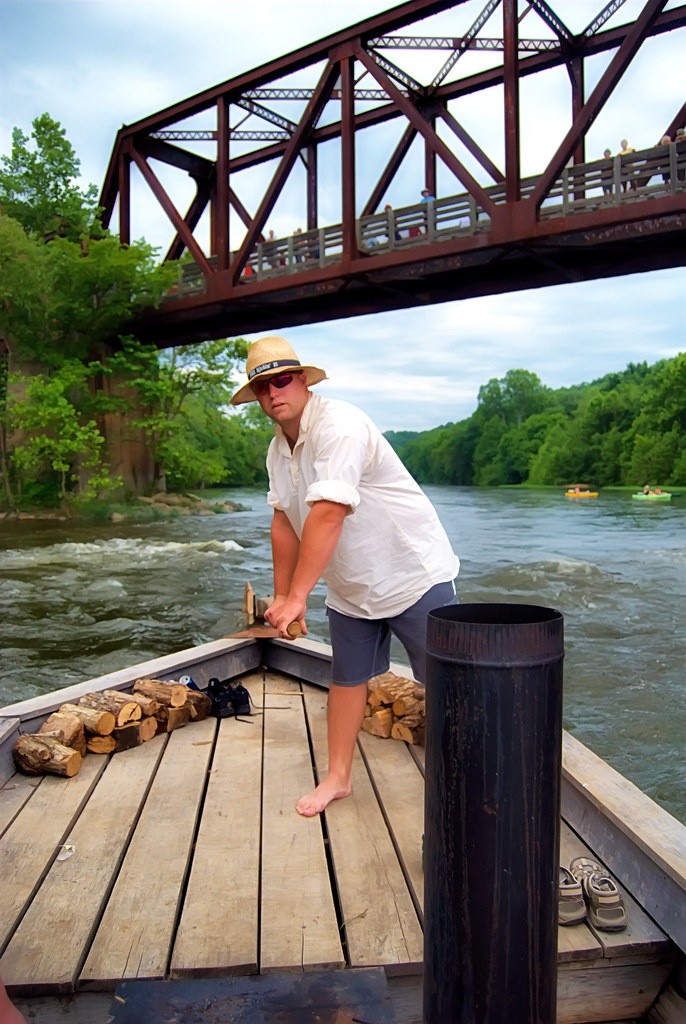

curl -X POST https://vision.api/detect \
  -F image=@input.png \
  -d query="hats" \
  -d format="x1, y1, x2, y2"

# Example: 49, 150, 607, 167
229, 337, 325, 406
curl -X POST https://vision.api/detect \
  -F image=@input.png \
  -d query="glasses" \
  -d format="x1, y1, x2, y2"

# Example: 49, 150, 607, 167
251, 369, 303, 396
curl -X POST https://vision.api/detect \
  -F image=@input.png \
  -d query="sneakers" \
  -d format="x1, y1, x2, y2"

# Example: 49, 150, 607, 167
204, 677, 290, 725
559, 857, 627, 932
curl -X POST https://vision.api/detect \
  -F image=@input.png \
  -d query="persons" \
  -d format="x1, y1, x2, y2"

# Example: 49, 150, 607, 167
230, 336, 460, 817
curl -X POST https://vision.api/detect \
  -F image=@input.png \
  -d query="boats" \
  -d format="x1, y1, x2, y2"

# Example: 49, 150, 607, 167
632, 491, 672, 502
564, 486, 599, 498
0, 584, 685, 1023
